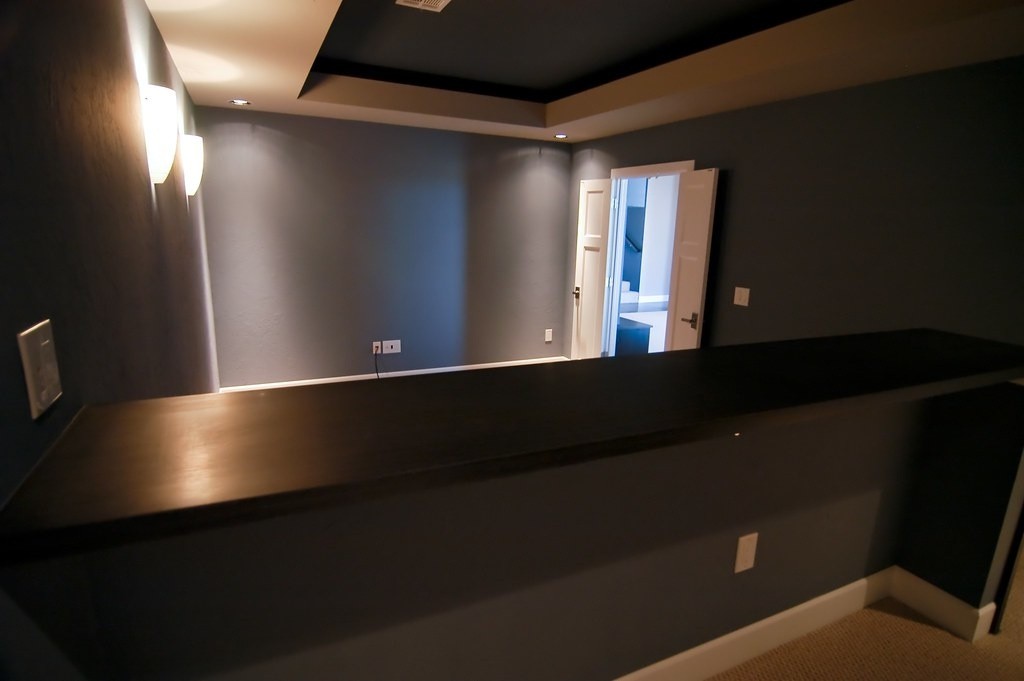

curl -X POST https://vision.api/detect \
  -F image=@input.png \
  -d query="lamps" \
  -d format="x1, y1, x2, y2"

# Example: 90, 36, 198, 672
184, 134, 205, 196
144, 85, 178, 184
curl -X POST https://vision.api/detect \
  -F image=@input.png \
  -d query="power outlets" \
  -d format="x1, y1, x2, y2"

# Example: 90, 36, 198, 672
373, 341, 382, 354
383, 340, 401, 354
